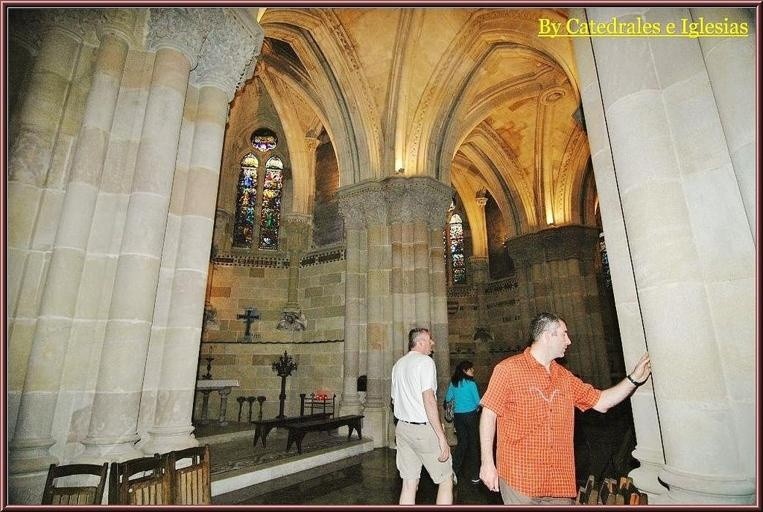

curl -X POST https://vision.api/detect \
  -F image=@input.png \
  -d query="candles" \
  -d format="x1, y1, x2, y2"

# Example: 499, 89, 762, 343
208, 346, 213, 357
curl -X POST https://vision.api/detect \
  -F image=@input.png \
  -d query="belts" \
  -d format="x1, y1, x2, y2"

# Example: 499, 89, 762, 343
396, 418, 427, 426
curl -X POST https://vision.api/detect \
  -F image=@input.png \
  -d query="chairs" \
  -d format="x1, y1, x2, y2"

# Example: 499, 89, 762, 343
574, 474, 649, 507
38, 441, 213, 505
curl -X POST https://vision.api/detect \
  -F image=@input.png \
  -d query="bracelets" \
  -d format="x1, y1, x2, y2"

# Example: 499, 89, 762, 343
627, 374, 640, 388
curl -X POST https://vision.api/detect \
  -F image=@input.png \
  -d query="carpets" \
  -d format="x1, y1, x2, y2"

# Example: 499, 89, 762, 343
209, 430, 358, 474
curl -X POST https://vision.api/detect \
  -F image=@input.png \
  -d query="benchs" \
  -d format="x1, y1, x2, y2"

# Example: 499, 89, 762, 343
284, 414, 365, 453
250, 412, 334, 449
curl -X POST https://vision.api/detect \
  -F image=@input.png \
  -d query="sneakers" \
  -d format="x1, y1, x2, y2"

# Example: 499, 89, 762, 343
471, 475, 481, 483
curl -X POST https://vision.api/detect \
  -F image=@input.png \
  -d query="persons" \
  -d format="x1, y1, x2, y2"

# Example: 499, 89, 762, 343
443, 358, 482, 487
476, 310, 653, 506
389, 327, 459, 505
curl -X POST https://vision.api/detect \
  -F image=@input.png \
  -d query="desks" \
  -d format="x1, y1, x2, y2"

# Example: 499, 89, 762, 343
196, 379, 241, 428
